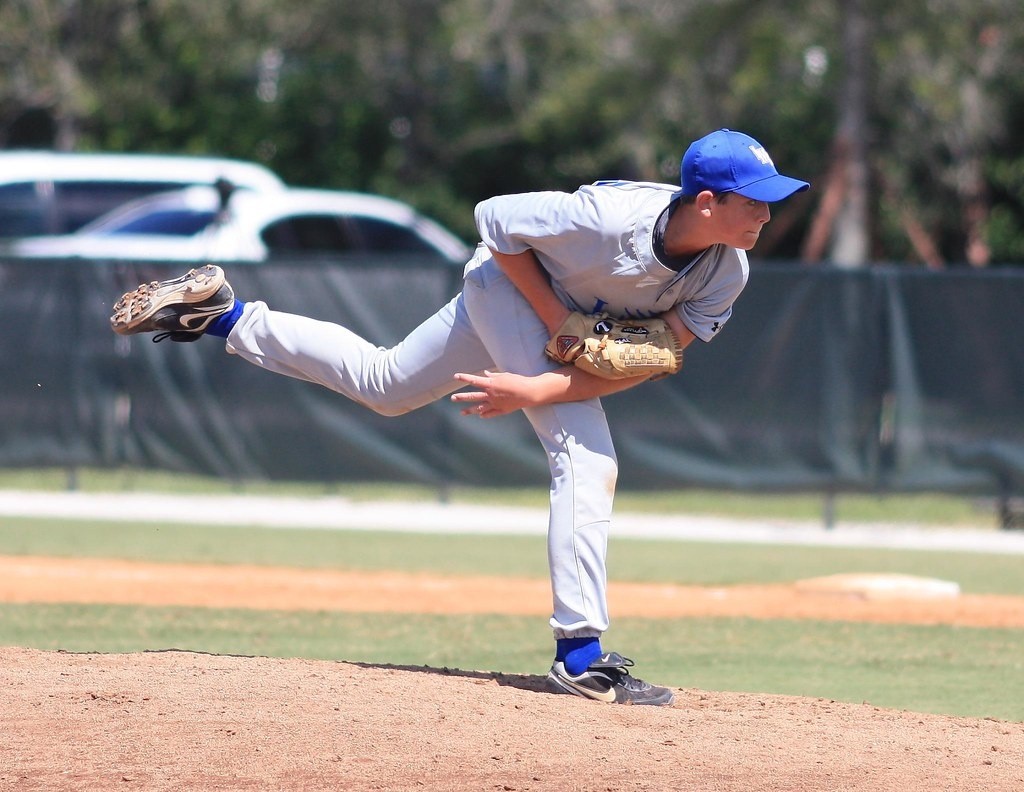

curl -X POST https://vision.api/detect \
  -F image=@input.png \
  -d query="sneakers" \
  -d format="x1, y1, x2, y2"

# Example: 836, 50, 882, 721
109, 265, 236, 343
544, 649, 673, 706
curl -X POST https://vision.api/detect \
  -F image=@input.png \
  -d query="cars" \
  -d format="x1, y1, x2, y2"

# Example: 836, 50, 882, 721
10, 187, 474, 266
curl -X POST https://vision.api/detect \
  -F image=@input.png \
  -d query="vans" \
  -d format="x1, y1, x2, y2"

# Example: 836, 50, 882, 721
1, 150, 284, 236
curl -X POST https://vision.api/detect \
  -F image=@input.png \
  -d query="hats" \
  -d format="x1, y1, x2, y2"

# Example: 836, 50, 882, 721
679, 128, 811, 204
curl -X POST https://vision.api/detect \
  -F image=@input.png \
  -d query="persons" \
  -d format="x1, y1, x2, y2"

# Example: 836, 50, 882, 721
109, 130, 811, 705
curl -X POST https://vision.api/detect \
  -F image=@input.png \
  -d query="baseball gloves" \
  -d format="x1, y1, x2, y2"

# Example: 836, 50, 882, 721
545, 311, 683, 380
